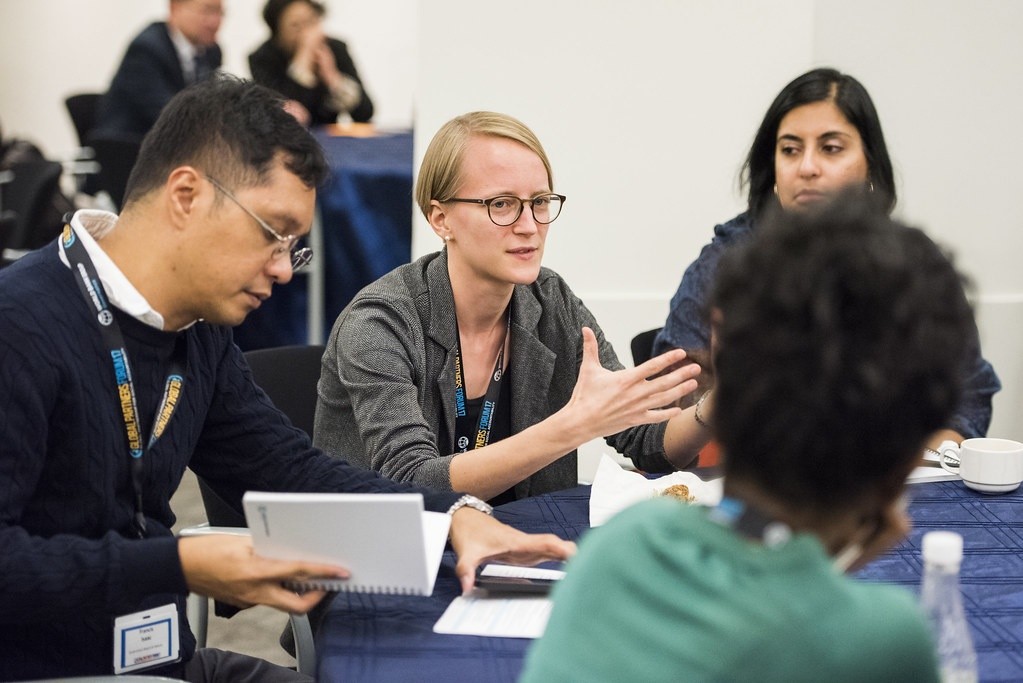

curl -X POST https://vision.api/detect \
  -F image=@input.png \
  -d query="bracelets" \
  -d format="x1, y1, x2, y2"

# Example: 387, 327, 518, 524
693, 390, 715, 434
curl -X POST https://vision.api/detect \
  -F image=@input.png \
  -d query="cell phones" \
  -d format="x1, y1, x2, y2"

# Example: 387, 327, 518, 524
474, 562, 555, 592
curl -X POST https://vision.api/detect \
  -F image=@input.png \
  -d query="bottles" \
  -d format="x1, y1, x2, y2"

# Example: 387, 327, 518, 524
920, 532, 979, 682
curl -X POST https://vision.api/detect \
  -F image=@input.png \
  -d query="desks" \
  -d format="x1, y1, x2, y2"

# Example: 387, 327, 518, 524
235, 122, 413, 357
284, 469, 1023, 683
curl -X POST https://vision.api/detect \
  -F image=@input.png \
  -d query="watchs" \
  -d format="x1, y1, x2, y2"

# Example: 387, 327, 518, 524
446, 493, 496, 515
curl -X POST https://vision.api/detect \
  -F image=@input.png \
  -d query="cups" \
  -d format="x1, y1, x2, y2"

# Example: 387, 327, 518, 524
939, 437, 1023, 494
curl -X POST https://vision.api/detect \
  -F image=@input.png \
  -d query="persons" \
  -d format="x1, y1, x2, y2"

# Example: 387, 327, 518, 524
92, 0, 375, 132
523, 200, 966, 683
312, 112, 715, 504
0, 76, 578, 683
653, 67, 1002, 458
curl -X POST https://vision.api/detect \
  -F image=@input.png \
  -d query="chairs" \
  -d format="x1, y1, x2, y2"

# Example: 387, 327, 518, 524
66, 93, 113, 214
195, 345, 331, 654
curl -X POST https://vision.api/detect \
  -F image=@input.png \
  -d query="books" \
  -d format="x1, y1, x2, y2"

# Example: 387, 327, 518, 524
241, 490, 450, 596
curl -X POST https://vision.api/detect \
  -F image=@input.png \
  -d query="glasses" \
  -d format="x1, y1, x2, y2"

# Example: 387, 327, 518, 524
435, 192, 566, 226
203, 174, 313, 273
